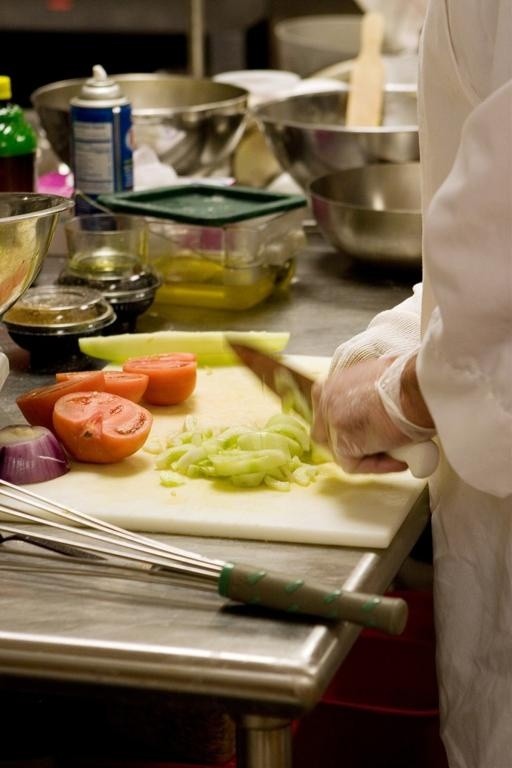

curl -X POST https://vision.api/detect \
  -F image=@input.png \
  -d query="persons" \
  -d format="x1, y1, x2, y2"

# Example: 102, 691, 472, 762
311, 0, 512, 768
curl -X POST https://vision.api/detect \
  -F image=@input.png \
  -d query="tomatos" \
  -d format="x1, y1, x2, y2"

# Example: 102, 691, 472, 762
15, 352, 197, 463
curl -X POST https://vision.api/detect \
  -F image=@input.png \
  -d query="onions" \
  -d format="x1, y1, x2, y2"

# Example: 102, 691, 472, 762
0, 425, 72, 485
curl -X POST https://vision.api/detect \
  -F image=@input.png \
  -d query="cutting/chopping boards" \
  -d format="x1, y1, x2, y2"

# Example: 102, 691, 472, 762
0, 353, 429, 550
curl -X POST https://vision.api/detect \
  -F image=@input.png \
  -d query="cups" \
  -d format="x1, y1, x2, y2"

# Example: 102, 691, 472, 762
63, 211, 150, 283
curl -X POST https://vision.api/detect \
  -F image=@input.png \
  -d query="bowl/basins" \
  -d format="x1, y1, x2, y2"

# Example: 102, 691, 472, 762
52, 276, 162, 334
2, 312, 119, 369
249, 88, 419, 198
306, 161, 422, 274
29, 70, 250, 180
0, 191, 78, 321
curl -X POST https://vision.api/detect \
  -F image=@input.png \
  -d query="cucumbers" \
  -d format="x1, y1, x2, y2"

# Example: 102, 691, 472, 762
79, 332, 289, 366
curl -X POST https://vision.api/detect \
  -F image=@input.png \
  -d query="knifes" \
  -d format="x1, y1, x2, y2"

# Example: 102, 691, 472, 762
227, 338, 440, 479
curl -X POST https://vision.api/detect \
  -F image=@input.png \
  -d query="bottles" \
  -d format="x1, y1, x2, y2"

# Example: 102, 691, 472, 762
67, 63, 136, 233
0, 75, 39, 193
